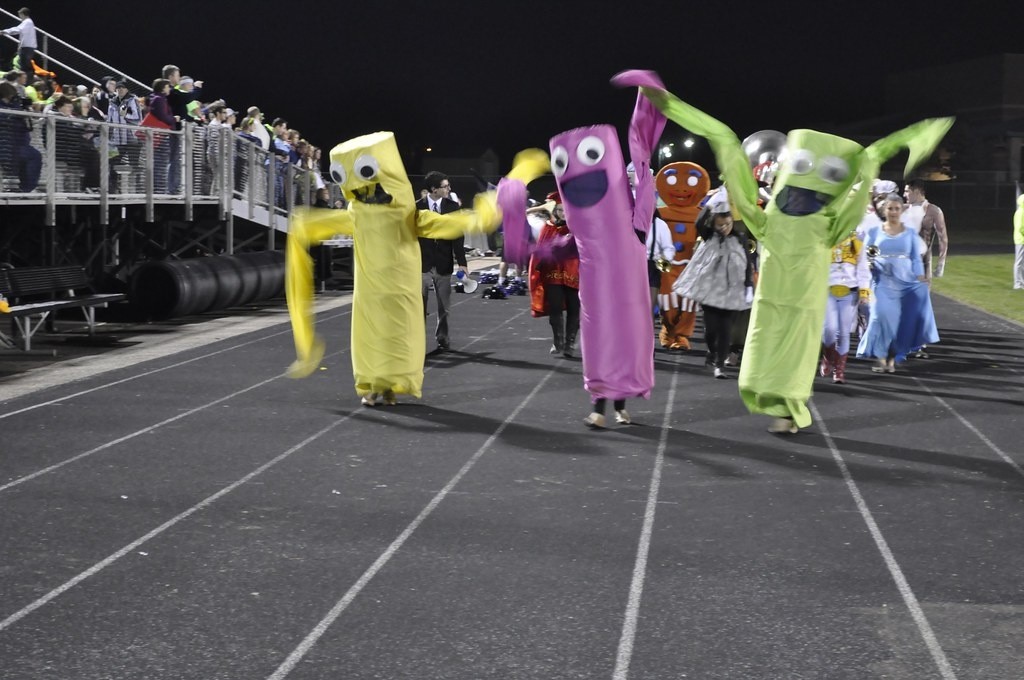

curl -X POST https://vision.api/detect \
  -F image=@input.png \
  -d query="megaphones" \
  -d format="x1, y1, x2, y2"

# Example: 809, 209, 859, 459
456, 271, 478, 294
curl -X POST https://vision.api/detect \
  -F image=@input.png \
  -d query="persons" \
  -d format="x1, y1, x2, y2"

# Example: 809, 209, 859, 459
282, 70, 958, 437
235, 106, 323, 214
1009, 194, 1024, 291
0, 7, 238, 197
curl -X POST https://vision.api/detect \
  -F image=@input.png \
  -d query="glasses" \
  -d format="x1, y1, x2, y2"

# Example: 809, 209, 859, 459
439, 184, 449, 187
556, 207, 563, 211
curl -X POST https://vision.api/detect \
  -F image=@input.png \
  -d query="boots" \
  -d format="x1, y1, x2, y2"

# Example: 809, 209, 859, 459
832, 350, 849, 384
819, 342, 836, 378
563, 316, 580, 356
549, 315, 563, 354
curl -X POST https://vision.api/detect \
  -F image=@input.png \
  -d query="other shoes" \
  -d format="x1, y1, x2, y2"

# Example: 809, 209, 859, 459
616, 409, 630, 423
706, 351, 713, 365
481, 284, 505, 299
768, 417, 799, 434
724, 352, 738, 366
506, 279, 526, 295
871, 364, 896, 373
584, 412, 606, 428
910, 350, 927, 359
714, 368, 726, 378
383, 391, 396, 405
361, 393, 376, 406
438, 338, 449, 348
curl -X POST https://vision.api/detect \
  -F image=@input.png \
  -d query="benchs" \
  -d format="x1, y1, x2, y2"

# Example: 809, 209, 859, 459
0, 263, 126, 356
0, 132, 134, 193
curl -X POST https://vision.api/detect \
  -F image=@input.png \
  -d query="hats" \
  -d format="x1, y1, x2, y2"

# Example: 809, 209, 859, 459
876, 179, 900, 193
226, 108, 238, 116
